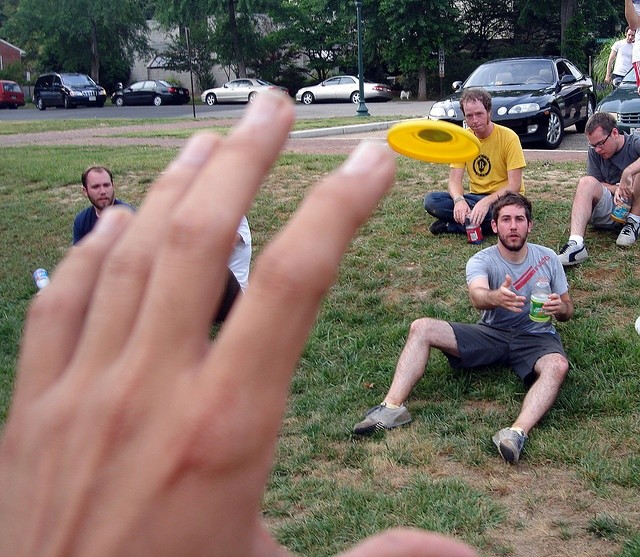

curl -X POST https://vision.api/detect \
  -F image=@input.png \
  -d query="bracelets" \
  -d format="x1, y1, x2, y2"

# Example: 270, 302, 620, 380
453, 195, 464, 204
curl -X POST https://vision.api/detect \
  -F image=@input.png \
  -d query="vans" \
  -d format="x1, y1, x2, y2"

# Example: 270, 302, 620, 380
32, 72, 106, 110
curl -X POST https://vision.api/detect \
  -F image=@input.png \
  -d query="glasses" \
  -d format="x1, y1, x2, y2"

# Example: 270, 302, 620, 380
588, 130, 612, 149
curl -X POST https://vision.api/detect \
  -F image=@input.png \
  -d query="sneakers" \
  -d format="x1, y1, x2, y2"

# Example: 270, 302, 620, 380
558, 240, 588, 266
615, 217, 640, 246
353, 403, 412, 435
429, 220, 448, 233
491, 427, 528, 463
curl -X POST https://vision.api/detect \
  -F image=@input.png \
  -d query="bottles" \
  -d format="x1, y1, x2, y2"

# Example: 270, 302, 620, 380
464, 211, 483, 244
33, 267, 49, 288
528, 275, 552, 322
610, 195, 632, 223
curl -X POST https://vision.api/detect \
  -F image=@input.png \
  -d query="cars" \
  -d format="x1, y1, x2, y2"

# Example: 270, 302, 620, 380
0, 80, 25, 109
201, 78, 288, 106
295, 76, 393, 105
427, 55, 597, 149
594, 65, 639, 132
110, 79, 190, 107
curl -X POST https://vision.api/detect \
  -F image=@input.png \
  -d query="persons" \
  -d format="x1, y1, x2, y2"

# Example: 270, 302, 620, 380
1, 86, 480, 556
557, 112, 639, 266
72, 164, 134, 244
603, 25, 636, 89
623, 0, 640, 92
351, 190, 576, 463
423, 84, 526, 235
208, 211, 252, 325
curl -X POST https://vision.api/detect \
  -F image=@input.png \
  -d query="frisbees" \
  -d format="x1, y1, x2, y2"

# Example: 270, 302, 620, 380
386, 119, 483, 163
